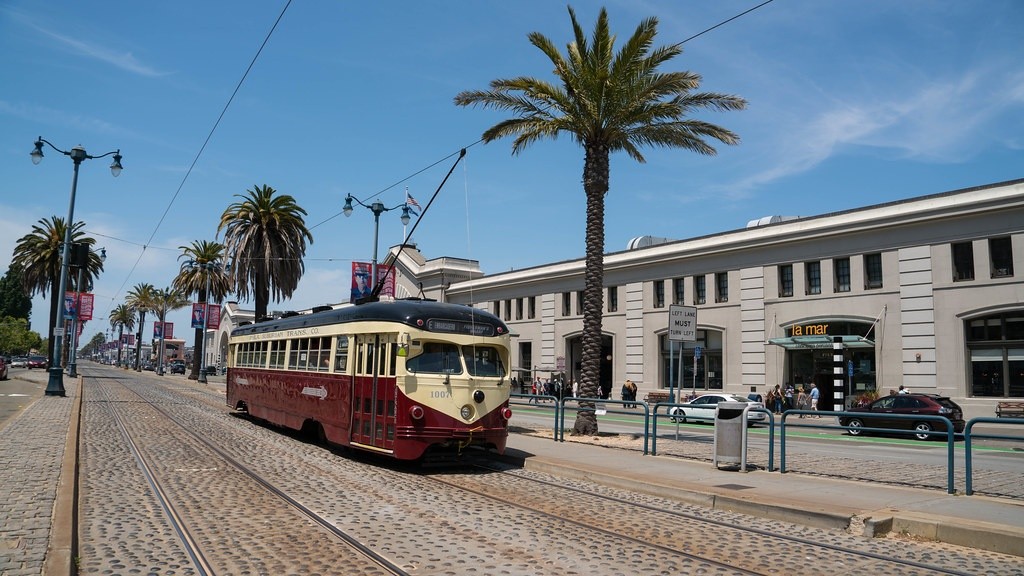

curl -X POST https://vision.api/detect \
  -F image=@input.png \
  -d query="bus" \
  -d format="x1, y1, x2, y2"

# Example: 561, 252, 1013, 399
227, 299, 511, 461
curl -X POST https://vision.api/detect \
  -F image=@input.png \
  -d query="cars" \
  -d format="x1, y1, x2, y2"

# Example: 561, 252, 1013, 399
206, 366, 216, 376
0, 359, 8, 380
11, 358, 28, 368
171, 360, 185, 375
29, 356, 49, 369
837, 393, 965, 441
94, 358, 166, 373
670, 395, 765, 428
0, 356, 20, 363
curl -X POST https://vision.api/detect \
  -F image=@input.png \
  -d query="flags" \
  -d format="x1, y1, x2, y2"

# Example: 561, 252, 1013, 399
405, 191, 423, 211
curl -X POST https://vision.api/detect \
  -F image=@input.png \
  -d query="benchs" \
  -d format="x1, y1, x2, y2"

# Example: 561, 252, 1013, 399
644, 392, 675, 403
996, 402, 1024, 418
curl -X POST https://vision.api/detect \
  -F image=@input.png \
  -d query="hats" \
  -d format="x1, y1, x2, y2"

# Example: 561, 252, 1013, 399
798, 386, 805, 391
811, 383, 816, 388
65, 295, 74, 301
193, 304, 202, 311
353, 263, 372, 275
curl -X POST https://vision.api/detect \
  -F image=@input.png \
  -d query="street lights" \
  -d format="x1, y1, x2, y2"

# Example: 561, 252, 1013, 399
157, 293, 167, 374
59, 246, 107, 378
198, 261, 214, 382
29, 135, 124, 396
342, 193, 411, 292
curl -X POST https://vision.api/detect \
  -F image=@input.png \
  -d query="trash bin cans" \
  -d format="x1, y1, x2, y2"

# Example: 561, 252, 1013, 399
716, 401, 748, 463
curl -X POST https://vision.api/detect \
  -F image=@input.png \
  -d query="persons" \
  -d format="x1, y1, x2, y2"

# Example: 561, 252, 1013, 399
620, 380, 639, 409
890, 385, 910, 394
689, 392, 697, 400
772, 383, 821, 418
509, 376, 603, 403
